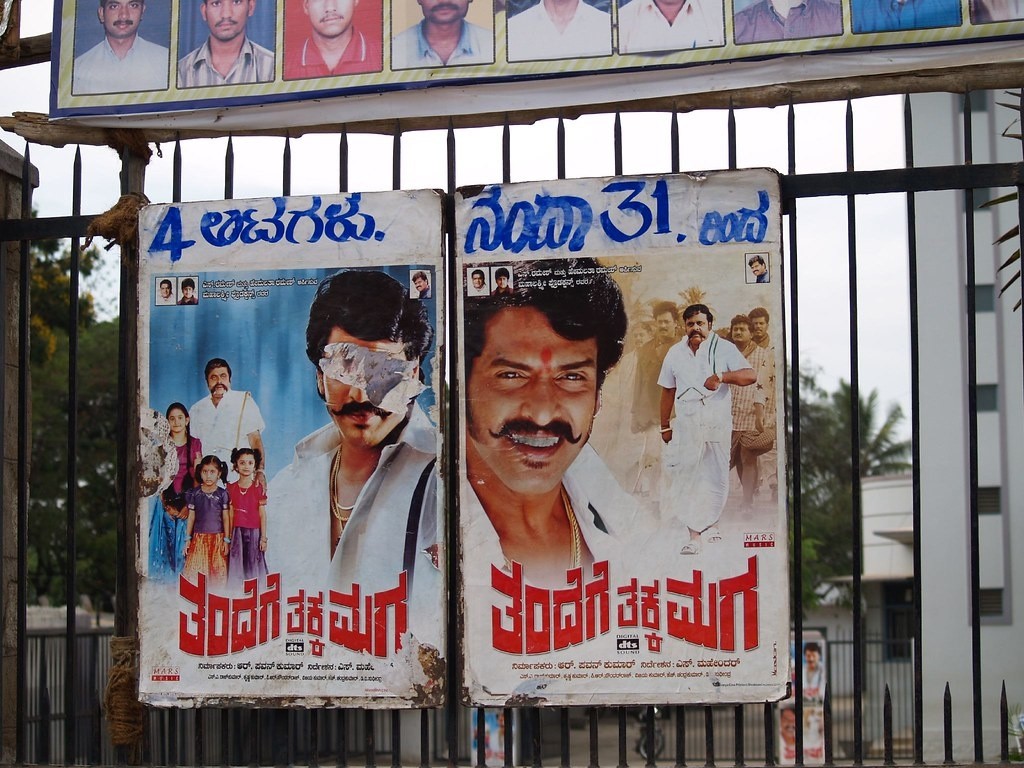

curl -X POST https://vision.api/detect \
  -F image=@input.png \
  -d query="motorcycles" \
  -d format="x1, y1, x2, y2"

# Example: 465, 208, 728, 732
637, 704, 665, 758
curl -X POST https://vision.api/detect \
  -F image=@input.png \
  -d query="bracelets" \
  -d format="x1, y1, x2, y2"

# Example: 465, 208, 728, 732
261, 536, 267, 542
257, 468, 264, 474
183, 535, 191, 541
661, 426, 671, 434
716, 371, 724, 383
224, 537, 230, 544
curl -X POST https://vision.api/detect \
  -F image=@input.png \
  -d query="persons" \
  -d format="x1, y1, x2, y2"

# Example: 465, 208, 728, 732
72, 0, 1023, 96
412, 271, 432, 299
781, 642, 822, 765
748, 256, 769, 282
157, 279, 175, 306
224, 446, 268, 588
178, 278, 200, 306
469, 270, 488, 296
492, 268, 513, 296
189, 358, 267, 495
182, 454, 232, 589
263, 268, 444, 695
595, 298, 776, 555
473, 712, 513, 758
462, 256, 687, 692
147, 403, 202, 580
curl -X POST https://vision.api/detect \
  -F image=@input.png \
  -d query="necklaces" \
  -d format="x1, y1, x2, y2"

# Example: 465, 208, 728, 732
238, 479, 252, 495
330, 449, 354, 545
504, 488, 583, 590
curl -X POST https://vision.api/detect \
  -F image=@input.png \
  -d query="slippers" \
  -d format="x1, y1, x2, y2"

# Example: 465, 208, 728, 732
680, 543, 697, 554
705, 527, 723, 543
